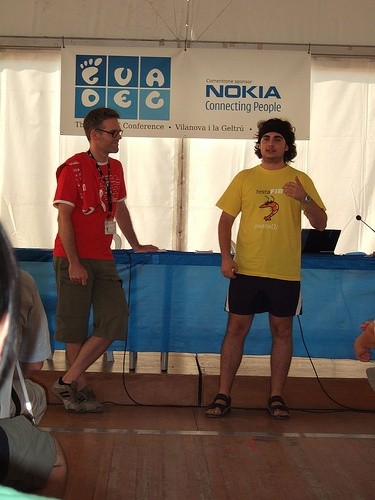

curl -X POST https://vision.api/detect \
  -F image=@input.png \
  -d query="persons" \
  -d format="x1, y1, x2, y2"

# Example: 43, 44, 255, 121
53, 107, 160, 413
0, 270, 52, 425
0, 218, 66, 496
205, 118, 328, 417
354, 320, 375, 392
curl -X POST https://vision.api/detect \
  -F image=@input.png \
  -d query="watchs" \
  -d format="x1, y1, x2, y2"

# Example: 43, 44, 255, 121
302, 194, 311, 204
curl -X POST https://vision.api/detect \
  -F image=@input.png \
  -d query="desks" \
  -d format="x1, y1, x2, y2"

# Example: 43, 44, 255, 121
12, 247, 375, 374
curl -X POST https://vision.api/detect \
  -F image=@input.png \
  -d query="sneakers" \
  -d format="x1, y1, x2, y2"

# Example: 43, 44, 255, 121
50, 376, 85, 414
76, 385, 105, 412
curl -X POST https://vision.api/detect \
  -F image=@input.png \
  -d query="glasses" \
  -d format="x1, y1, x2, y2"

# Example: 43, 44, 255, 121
95, 128, 124, 138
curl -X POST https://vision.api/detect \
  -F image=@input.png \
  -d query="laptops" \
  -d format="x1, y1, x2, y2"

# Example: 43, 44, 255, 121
301, 228, 341, 255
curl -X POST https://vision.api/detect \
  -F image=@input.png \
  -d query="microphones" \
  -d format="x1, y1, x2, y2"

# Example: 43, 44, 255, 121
356, 215, 375, 232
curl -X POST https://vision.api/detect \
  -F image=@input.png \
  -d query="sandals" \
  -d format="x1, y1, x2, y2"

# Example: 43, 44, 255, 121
267, 396, 291, 418
204, 393, 232, 417
